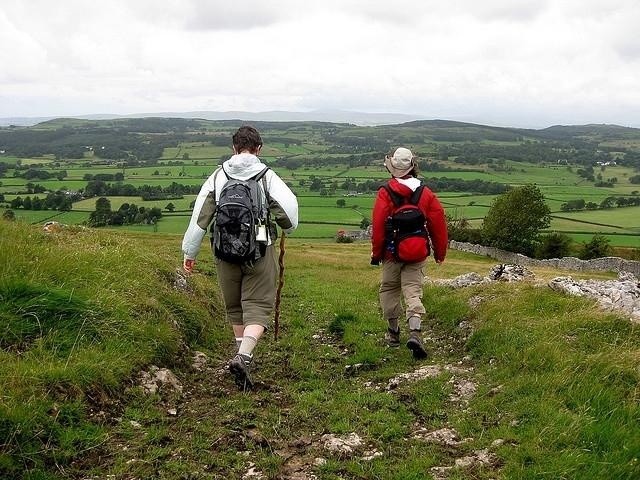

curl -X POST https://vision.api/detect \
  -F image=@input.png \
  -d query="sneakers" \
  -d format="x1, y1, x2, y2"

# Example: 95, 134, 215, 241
406, 332, 428, 359
383, 326, 401, 347
228, 353, 256, 392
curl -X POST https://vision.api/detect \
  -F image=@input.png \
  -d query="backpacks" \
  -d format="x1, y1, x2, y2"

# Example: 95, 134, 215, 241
376, 181, 431, 266
209, 167, 271, 276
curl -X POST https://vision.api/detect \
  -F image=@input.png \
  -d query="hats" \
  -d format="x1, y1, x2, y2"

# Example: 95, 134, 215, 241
384, 146, 419, 178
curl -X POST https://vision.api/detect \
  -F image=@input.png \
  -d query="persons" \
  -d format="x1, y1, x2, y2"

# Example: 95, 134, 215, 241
182, 125, 299, 391
369, 147, 449, 358
